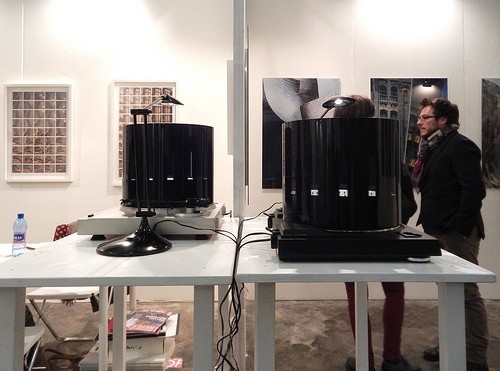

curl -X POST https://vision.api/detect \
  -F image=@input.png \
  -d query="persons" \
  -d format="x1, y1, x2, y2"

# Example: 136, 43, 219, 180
332, 95, 418, 371
412, 98, 492, 371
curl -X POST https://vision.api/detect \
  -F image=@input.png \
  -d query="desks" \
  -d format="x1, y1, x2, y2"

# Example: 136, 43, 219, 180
0, 218, 240, 371
235, 216, 497, 371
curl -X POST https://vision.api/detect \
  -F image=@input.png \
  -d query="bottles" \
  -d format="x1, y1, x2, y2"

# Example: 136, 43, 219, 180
12, 213, 27, 256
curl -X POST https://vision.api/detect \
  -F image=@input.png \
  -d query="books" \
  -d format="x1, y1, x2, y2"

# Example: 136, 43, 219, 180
126, 308, 173, 335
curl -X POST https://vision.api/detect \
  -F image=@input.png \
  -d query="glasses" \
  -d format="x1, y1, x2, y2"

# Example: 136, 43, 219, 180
417, 115, 436, 122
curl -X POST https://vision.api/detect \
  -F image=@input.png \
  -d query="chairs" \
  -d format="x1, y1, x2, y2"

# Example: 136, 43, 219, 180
25, 221, 114, 371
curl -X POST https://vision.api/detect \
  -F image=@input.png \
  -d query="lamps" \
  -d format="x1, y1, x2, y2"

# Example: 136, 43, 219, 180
96, 93, 184, 257
320, 97, 356, 118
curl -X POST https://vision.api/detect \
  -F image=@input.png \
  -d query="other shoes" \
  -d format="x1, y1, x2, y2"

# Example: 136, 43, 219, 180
423, 345, 439, 361
381, 355, 423, 371
467, 362, 490, 371
345, 357, 376, 371
